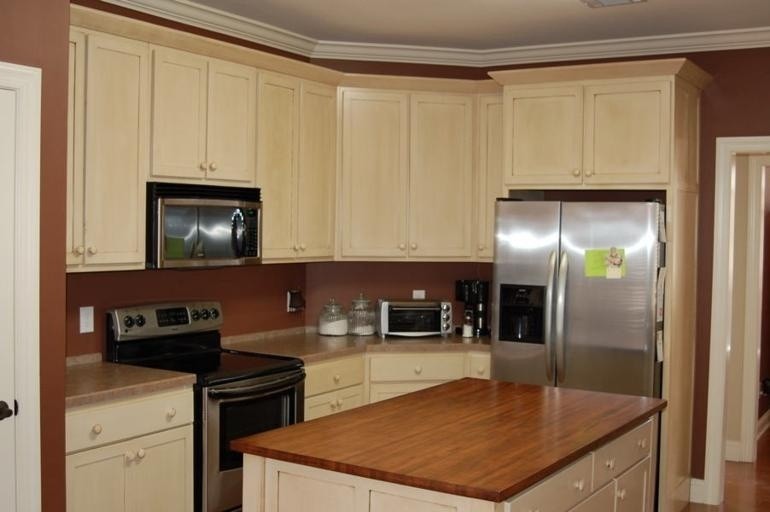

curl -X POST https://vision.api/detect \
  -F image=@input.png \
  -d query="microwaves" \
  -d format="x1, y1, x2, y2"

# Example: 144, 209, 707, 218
151, 195, 263, 269
374, 296, 454, 340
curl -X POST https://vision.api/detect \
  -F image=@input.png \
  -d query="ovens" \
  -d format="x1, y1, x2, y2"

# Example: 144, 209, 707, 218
198, 367, 306, 512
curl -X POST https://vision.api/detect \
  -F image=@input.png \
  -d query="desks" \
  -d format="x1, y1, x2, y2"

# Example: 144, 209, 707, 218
237, 375, 667, 512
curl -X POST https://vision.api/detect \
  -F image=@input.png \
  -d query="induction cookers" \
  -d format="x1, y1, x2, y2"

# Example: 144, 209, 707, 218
117, 348, 305, 385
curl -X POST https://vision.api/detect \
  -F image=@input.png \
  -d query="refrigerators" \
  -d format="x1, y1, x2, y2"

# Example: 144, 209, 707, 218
488, 199, 666, 403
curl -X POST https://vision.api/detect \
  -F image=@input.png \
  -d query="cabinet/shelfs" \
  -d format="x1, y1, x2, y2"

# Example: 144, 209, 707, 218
368, 354, 466, 405
256, 48, 345, 263
304, 354, 367, 425
63, 3, 148, 272
336, 68, 479, 263
147, 21, 259, 191
64, 384, 200, 510
466, 354, 491, 381
483, 58, 711, 192
476, 79, 501, 263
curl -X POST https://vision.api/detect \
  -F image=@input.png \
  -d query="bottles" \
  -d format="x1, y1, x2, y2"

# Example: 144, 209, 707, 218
317, 296, 349, 336
349, 292, 377, 336
462, 281, 487, 338
